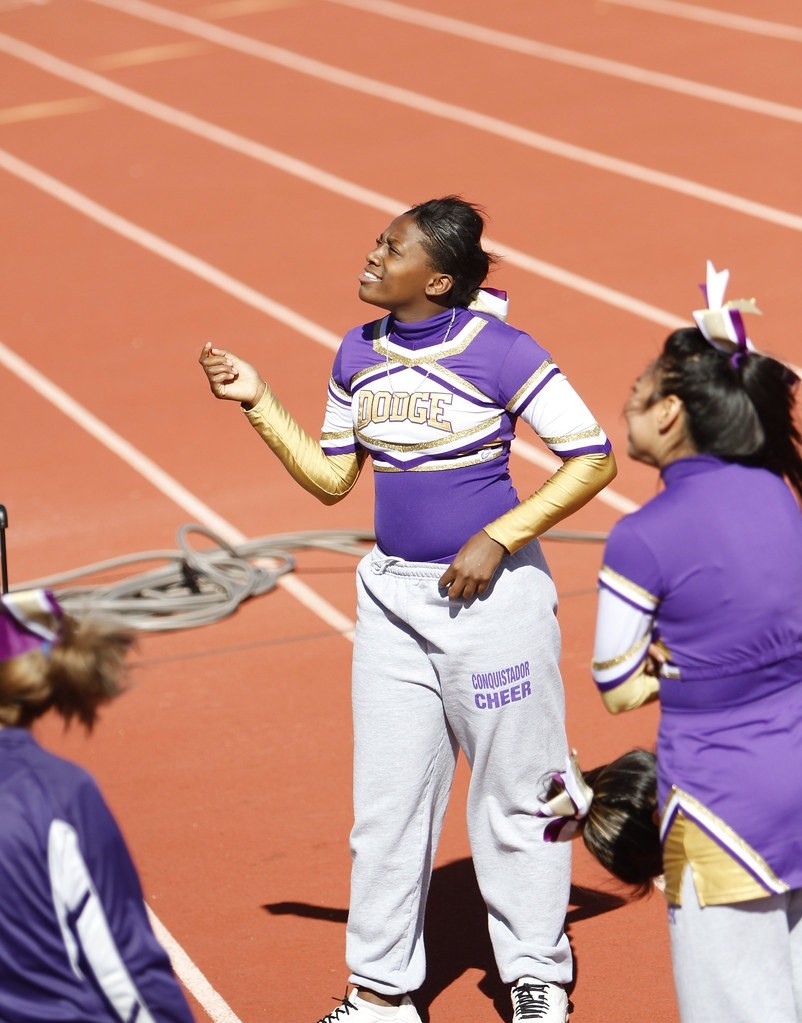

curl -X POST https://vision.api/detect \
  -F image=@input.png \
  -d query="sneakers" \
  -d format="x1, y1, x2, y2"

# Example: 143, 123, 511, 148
511, 978, 569, 1023
317, 985, 422, 1023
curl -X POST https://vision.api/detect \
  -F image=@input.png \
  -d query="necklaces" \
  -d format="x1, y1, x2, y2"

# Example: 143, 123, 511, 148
386, 306, 455, 399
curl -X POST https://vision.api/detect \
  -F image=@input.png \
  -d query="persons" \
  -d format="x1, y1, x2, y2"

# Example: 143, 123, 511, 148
591, 258, 802, 1023
0, 590, 195, 1023
199, 194, 618, 1023
535, 746, 664, 901
